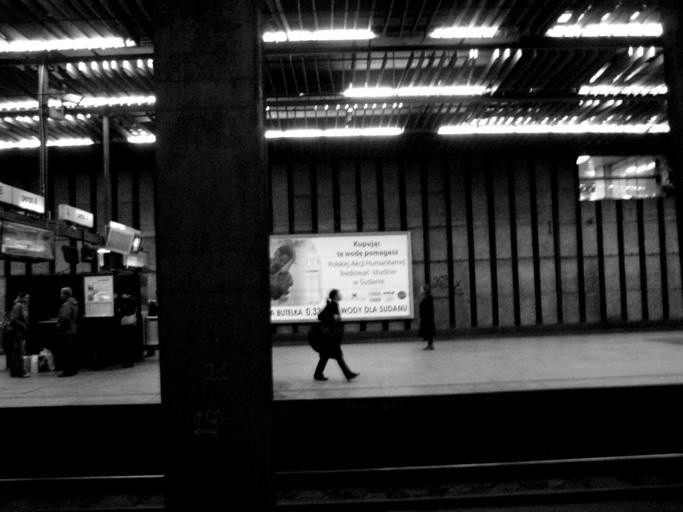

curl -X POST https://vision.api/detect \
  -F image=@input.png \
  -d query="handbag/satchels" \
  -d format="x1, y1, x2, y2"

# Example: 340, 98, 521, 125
306, 321, 323, 353
121, 313, 137, 327
0, 314, 15, 332
320, 322, 344, 335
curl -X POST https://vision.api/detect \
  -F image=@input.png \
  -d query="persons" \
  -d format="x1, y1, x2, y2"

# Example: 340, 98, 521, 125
116, 288, 142, 369
143, 297, 159, 360
417, 283, 436, 350
268, 242, 295, 300
52, 287, 80, 377
305, 288, 359, 382
0, 290, 30, 377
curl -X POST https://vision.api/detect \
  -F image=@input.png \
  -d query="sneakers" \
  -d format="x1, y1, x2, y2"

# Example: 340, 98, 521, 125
58, 370, 77, 378
423, 346, 432, 350
314, 375, 328, 381
11, 372, 31, 378
345, 373, 358, 379
123, 362, 134, 368
